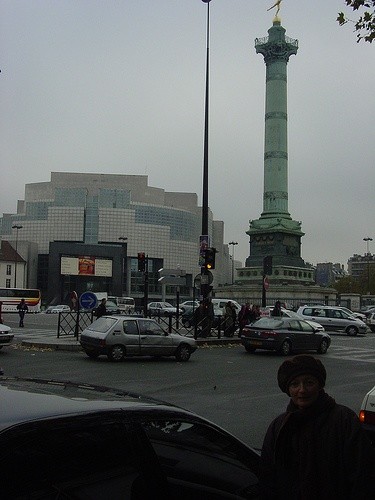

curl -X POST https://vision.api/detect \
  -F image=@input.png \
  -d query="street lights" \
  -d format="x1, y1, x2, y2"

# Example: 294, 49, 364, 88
363, 237, 374, 294
228, 241, 238, 286
11, 225, 24, 288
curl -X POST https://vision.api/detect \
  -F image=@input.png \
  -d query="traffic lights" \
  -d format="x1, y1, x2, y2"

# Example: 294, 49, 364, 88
204, 247, 215, 270
137, 252, 145, 272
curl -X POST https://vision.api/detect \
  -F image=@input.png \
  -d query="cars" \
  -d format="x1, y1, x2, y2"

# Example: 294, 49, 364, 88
314, 305, 375, 334
181, 307, 226, 328
259, 303, 325, 332
97, 300, 121, 315
240, 316, 331, 357
0, 319, 14, 351
147, 302, 183, 317
178, 300, 201, 311
359, 385, 375, 421
46, 305, 70, 314
0, 375, 263, 500
79, 315, 198, 363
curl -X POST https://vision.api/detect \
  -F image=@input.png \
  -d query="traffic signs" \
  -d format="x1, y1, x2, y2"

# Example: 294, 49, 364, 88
158, 276, 186, 286
157, 268, 186, 277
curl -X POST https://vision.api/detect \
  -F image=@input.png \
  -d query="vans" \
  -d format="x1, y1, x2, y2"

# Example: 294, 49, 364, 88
210, 299, 241, 316
296, 305, 367, 337
106, 296, 136, 313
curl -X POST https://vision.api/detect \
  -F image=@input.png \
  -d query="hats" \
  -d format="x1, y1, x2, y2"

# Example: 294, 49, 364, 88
278, 355, 327, 398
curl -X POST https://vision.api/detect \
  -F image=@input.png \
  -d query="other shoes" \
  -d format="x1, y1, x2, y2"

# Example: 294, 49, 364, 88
19, 325, 24, 327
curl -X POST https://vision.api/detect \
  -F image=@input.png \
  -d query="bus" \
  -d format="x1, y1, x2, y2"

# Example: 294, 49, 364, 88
0, 287, 42, 314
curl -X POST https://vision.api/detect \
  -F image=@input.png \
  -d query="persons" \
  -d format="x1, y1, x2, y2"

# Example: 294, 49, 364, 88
16, 298, 29, 328
95, 298, 108, 320
270, 300, 282, 317
193, 298, 262, 340
258, 353, 375, 500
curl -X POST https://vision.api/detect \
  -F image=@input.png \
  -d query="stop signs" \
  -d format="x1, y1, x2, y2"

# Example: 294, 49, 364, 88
264, 276, 269, 290
